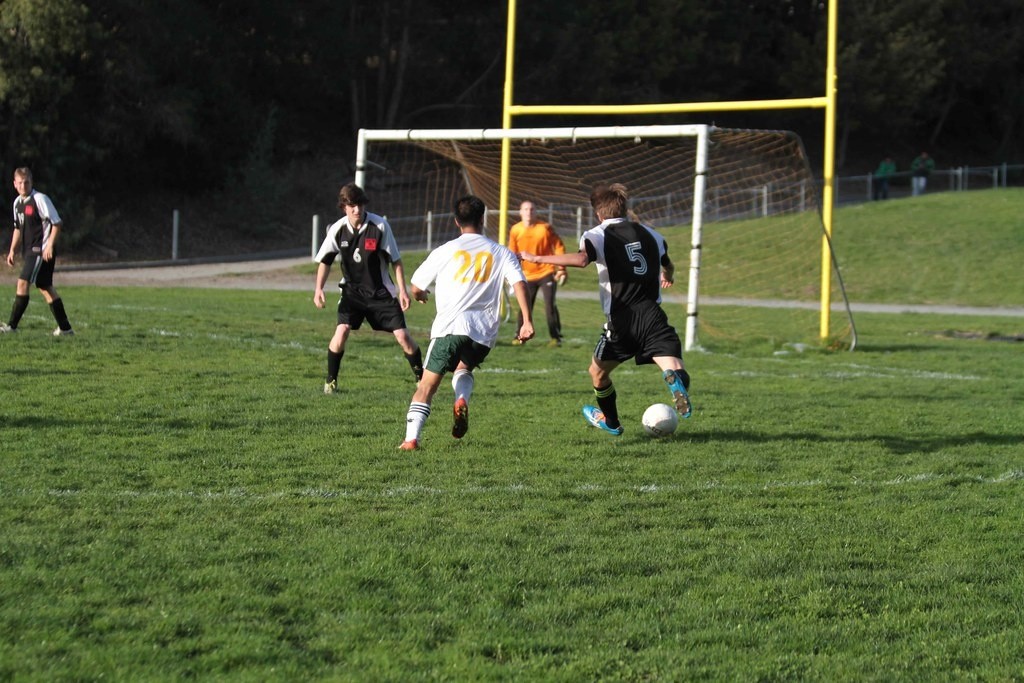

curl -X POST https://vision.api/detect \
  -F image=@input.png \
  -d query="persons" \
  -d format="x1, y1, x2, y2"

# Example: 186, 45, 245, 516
508, 201, 568, 347
398, 194, 535, 452
0, 168, 75, 336
872, 156, 896, 199
515, 181, 692, 435
910, 152, 935, 196
314, 184, 424, 393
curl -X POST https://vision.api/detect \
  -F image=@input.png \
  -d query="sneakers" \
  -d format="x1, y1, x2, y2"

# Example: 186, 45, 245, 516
53, 327, 74, 336
582, 406, 624, 436
452, 398, 470, 439
548, 338, 562, 349
324, 378, 338, 397
512, 339, 525, 345
662, 369, 692, 419
0, 325, 19, 333
394, 439, 420, 451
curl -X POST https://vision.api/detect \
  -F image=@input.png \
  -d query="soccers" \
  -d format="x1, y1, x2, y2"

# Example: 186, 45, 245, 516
641, 403, 678, 437
